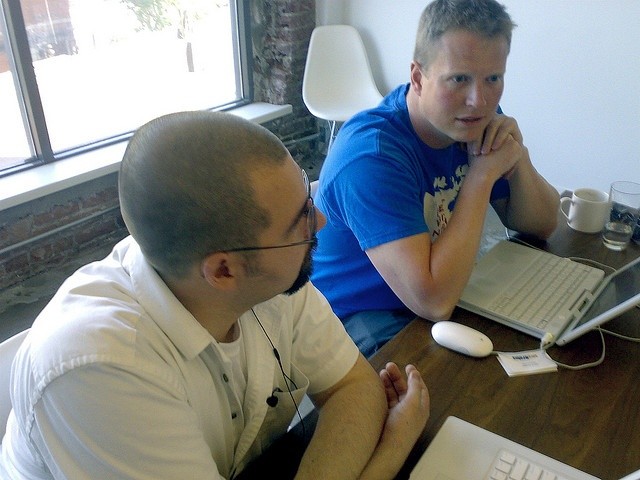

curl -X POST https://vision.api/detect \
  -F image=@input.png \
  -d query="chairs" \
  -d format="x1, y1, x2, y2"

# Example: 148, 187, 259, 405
302, 23, 384, 153
0, 324, 30, 438
310, 181, 323, 201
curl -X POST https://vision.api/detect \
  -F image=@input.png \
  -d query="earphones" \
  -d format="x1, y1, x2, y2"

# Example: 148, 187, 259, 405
267, 394, 279, 407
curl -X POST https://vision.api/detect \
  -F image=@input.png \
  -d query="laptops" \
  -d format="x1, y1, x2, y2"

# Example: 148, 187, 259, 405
407, 415, 640, 480
453, 239, 640, 347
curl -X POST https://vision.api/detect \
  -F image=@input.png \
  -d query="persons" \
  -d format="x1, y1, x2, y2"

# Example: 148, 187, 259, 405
0, 109, 432, 476
311, 0, 561, 359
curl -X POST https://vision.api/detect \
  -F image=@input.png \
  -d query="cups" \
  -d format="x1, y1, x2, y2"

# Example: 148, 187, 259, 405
601, 180, 640, 252
559, 187, 609, 234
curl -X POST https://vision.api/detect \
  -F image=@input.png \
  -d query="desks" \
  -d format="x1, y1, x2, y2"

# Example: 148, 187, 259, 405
233, 190, 640, 479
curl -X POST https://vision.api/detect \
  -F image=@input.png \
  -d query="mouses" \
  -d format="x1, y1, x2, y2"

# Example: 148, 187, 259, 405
430, 320, 493, 357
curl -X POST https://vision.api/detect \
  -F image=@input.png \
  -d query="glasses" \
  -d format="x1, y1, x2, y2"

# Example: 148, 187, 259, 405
201, 168, 316, 280
